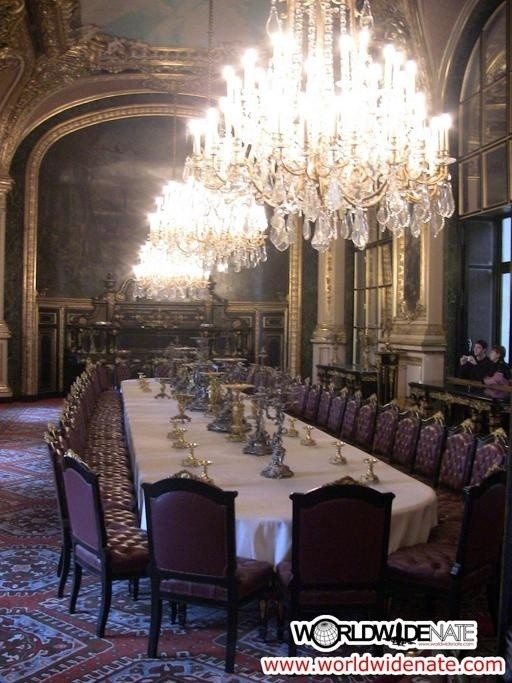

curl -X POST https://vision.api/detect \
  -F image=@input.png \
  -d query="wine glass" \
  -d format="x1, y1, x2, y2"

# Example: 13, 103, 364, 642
198, 458, 214, 486
167, 421, 181, 439
174, 427, 188, 448
329, 441, 347, 464
135, 371, 152, 393
301, 425, 316, 446
360, 457, 381, 483
180, 441, 199, 467
287, 417, 298, 436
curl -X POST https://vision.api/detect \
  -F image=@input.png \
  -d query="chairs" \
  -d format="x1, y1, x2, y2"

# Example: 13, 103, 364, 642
289, 475, 396, 656
143, 477, 250, 675
45, 356, 147, 637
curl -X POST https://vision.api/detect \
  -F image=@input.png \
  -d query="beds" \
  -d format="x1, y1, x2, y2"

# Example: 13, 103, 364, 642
120, 373, 440, 623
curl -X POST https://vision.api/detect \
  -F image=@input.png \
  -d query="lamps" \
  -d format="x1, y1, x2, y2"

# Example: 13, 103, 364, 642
183, 0, 456, 253
132, 241, 215, 305
145, 68, 267, 271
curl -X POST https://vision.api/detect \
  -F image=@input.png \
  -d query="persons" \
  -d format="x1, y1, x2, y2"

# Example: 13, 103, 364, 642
484, 345, 510, 399
459, 341, 490, 397
